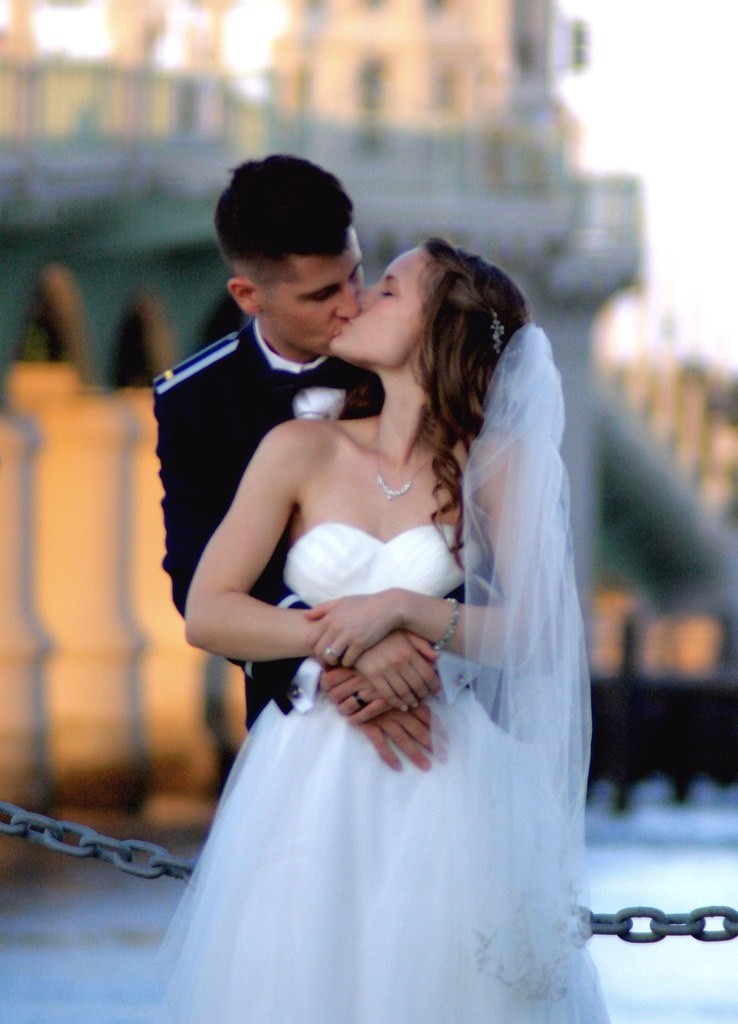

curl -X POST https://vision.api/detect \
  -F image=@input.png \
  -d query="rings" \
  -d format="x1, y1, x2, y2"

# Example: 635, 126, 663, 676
354, 692, 368, 708
326, 647, 341, 658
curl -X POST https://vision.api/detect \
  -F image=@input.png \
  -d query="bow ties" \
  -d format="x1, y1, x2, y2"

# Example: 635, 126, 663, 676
261, 355, 358, 413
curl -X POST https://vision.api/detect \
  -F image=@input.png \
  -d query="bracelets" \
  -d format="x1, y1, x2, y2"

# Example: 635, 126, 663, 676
434, 598, 461, 651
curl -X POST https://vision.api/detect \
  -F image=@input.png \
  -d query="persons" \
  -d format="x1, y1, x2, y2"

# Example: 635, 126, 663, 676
153, 154, 502, 774
124, 237, 610, 1024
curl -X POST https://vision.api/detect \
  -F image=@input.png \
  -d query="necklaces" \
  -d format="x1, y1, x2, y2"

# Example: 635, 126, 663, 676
376, 415, 430, 500
